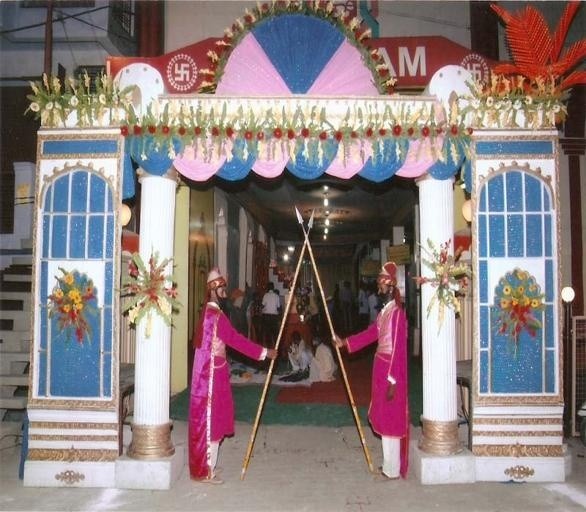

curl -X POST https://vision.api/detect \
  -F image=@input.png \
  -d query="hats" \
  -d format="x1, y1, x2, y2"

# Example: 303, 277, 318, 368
206, 270, 227, 290
376, 261, 398, 287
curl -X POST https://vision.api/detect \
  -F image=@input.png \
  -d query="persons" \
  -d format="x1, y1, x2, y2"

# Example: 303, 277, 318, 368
188, 266, 280, 484
332, 260, 409, 483
228, 280, 383, 382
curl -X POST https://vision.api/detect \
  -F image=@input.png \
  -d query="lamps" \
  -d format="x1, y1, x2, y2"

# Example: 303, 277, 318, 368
121, 203, 131, 227
322, 184, 329, 241
460, 192, 472, 223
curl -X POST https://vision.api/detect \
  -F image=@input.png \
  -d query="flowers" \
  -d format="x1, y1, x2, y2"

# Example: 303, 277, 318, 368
111, 242, 184, 339
38, 265, 101, 348
489, 269, 548, 357
456, 66, 573, 131
195, 0, 397, 96
112, 100, 475, 168
21, 63, 140, 130
409, 238, 477, 337
